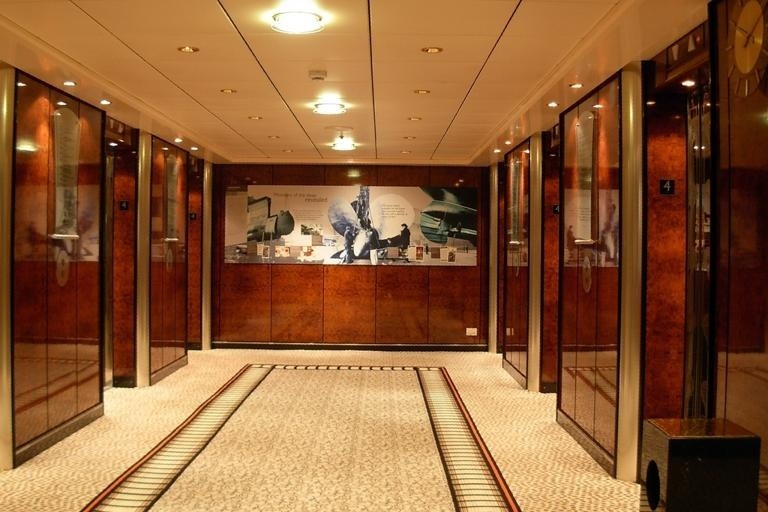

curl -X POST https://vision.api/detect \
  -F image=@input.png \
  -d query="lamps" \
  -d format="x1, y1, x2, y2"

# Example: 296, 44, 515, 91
313, 103, 346, 114
270, 12, 325, 35
331, 144, 356, 150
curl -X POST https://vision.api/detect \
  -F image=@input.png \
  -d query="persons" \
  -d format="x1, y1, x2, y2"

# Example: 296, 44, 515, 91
566, 225, 576, 264
342, 226, 355, 263
399, 223, 411, 262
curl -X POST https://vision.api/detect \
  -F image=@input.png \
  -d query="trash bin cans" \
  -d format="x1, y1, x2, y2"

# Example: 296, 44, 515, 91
639, 418, 761, 512
